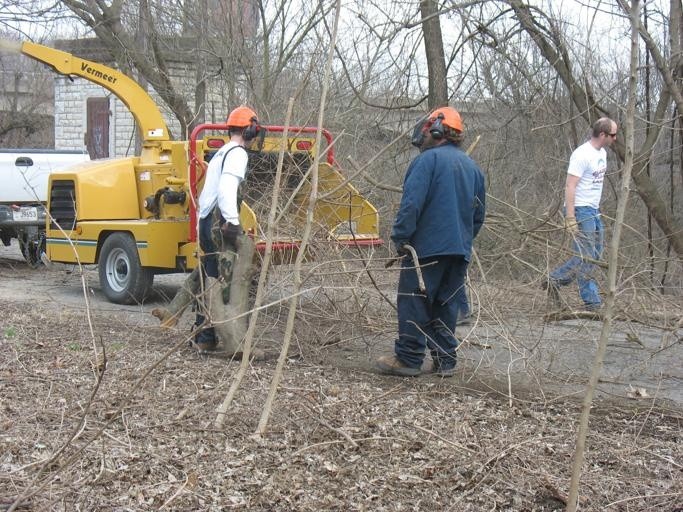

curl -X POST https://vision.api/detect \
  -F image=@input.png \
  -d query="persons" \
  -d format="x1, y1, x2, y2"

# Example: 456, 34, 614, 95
375, 106, 486, 378
188, 106, 267, 349
540, 117, 618, 313
455, 293, 471, 325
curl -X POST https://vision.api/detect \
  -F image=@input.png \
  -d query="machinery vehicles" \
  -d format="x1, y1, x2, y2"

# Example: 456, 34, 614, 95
2, 42, 384, 306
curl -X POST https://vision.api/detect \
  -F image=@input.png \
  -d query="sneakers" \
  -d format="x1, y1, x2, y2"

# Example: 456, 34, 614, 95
376, 354, 422, 377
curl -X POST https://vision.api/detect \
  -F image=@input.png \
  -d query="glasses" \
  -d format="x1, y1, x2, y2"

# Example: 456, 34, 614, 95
600, 131, 616, 137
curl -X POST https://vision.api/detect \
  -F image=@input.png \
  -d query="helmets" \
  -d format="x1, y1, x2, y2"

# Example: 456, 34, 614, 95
427, 107, 463, 134
226, 106, 259, 127
